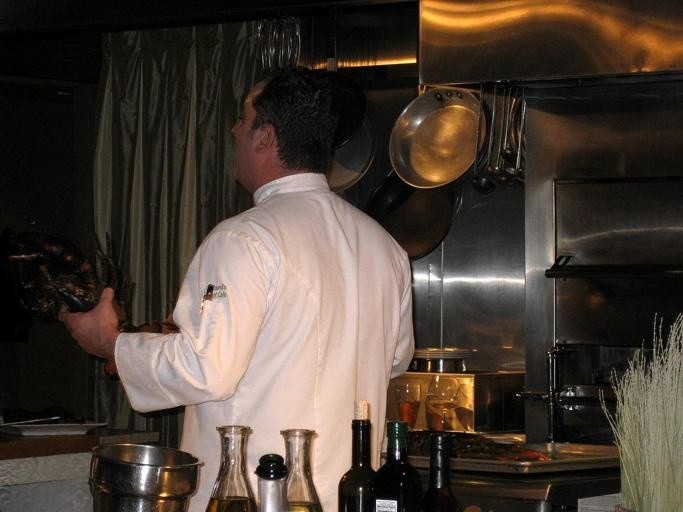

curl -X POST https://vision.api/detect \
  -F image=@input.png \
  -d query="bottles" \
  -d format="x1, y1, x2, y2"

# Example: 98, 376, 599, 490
204, 418, 467, 512
258, 17, 303, 72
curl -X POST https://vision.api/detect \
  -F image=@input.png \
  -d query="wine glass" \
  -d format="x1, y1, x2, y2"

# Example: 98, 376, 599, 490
395, 377, 461, 443
47, 230, 158, 382
9, 238, 72, 356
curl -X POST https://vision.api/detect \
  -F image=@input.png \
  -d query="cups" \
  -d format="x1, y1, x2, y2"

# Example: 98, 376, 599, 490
89, 442, 203, 512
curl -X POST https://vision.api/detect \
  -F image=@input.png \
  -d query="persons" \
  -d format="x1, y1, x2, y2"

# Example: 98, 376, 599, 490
58, 66, 417, 511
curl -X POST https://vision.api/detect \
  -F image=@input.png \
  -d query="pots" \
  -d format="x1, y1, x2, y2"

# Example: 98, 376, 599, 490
329, 82, 465, 260
388, 86, 489, 194
